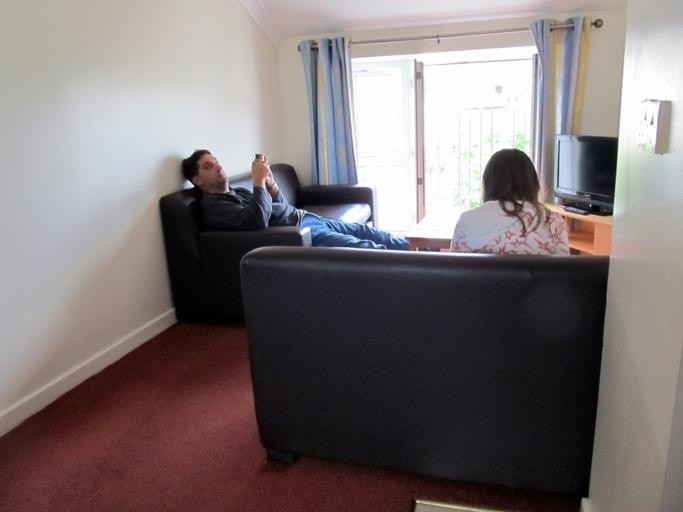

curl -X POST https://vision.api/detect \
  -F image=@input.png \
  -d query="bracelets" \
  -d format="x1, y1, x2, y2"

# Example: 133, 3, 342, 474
267, 182, 275, 191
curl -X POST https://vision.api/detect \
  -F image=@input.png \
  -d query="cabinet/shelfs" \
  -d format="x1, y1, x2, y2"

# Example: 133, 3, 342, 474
542, 203, 613, 258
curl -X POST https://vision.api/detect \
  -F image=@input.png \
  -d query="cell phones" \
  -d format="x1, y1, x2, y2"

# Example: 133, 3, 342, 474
255, 153, 262, 159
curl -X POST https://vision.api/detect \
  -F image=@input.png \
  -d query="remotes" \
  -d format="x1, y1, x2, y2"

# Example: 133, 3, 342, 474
563, 206, 589, 216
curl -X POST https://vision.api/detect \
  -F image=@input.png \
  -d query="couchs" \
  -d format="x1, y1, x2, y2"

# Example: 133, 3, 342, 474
158, 163, 378, 327
239, 243, 611, 500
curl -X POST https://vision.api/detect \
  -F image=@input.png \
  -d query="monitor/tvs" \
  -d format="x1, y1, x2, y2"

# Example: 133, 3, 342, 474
552, 134, 619, 216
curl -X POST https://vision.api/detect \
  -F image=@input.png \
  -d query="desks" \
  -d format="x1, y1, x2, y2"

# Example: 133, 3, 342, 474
405, 215, 450, 251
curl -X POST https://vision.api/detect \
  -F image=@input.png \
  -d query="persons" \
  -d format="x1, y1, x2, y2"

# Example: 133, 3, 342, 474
180, 148, 411, 249
449, 148, 570, 256
